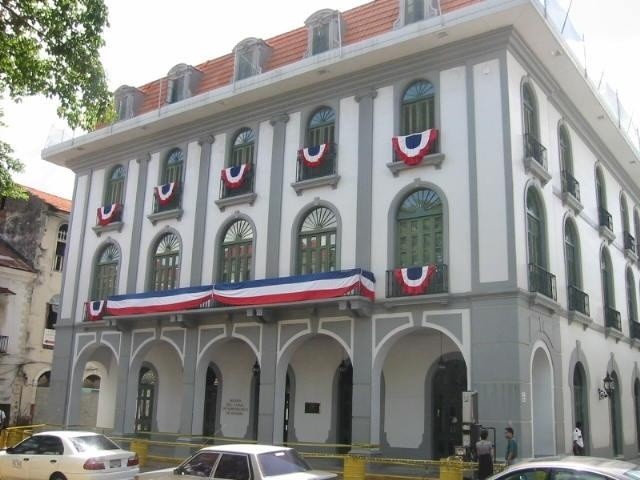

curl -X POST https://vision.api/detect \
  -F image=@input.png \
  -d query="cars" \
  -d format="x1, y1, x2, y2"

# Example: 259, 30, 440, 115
485, 456, 640, 480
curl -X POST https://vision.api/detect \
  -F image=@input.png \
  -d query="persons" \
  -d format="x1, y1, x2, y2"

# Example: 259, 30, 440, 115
573, 422, 584, 455
504, 428, 518, 466
475, 430, 494, 480
